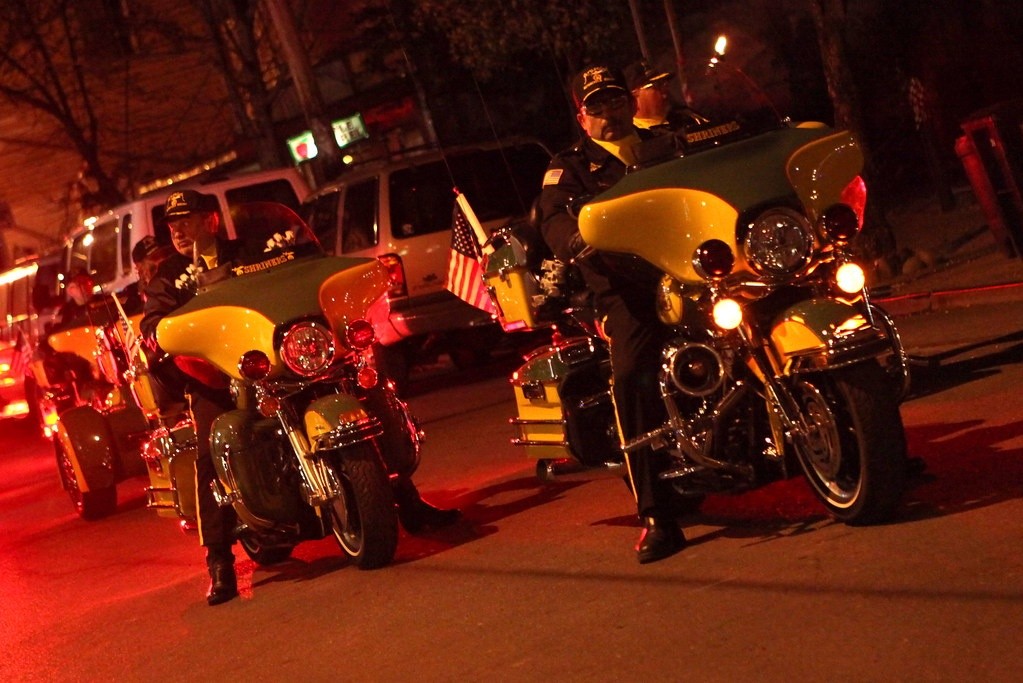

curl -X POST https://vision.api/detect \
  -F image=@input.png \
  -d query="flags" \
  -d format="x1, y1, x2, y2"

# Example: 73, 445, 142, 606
442, 202, 501, 315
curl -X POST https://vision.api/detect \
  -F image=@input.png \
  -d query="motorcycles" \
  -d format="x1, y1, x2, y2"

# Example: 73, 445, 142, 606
34, 245, 425, 566
483, 122, 913, 528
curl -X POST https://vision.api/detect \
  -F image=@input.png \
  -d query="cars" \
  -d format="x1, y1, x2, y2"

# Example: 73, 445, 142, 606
303, 136, 561, 387
0, 250, 66, 422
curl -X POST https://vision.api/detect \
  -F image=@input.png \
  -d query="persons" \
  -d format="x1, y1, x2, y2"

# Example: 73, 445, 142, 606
541, 68, 690, 562
140, 190, 460, 606
52, 267, 97, 325
123, 235, 163, 320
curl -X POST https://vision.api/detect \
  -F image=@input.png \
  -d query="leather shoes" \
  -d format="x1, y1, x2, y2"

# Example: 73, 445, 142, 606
634, 513, 685, 561
909, 456, 927, 475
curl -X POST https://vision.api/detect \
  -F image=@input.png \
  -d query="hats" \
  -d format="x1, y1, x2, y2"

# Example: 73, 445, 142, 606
628, 66, 676, 92
160, 190, 208, 223
66, 266, 94, 285
572, 65, 631, 109
132, 235, 165, 263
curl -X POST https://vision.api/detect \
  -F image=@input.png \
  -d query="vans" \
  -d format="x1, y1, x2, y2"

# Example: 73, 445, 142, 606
58, 166, 312, 306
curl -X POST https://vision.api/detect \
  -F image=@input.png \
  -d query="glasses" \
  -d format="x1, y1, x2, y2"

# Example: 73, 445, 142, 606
580, 96, 629, 116
632, 80, 670, 91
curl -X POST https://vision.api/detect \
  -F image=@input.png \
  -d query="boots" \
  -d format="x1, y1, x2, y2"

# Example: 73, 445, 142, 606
206, 554, 237, 605
395, 480, 462, 535
